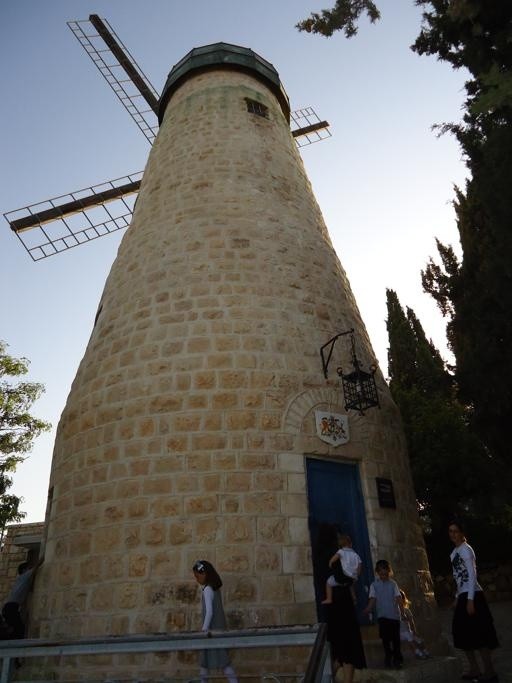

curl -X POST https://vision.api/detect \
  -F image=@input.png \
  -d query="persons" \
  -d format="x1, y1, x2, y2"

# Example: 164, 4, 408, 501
443, 519, 501, 683
192, 560, 241, 682
396, 588, 433, 661
1, 553, 45, 670
319, 534, 365, 605
312, 523, 368, 682
360, 558, 408, 669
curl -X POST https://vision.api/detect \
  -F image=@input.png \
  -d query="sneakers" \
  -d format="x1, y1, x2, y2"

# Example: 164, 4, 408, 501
384, 663, 404, 670
416, 651, 433, 660
461, 671, 499, 683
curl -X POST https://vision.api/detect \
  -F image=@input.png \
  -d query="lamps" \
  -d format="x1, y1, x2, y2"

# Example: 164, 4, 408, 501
320, 327, 381, 415
